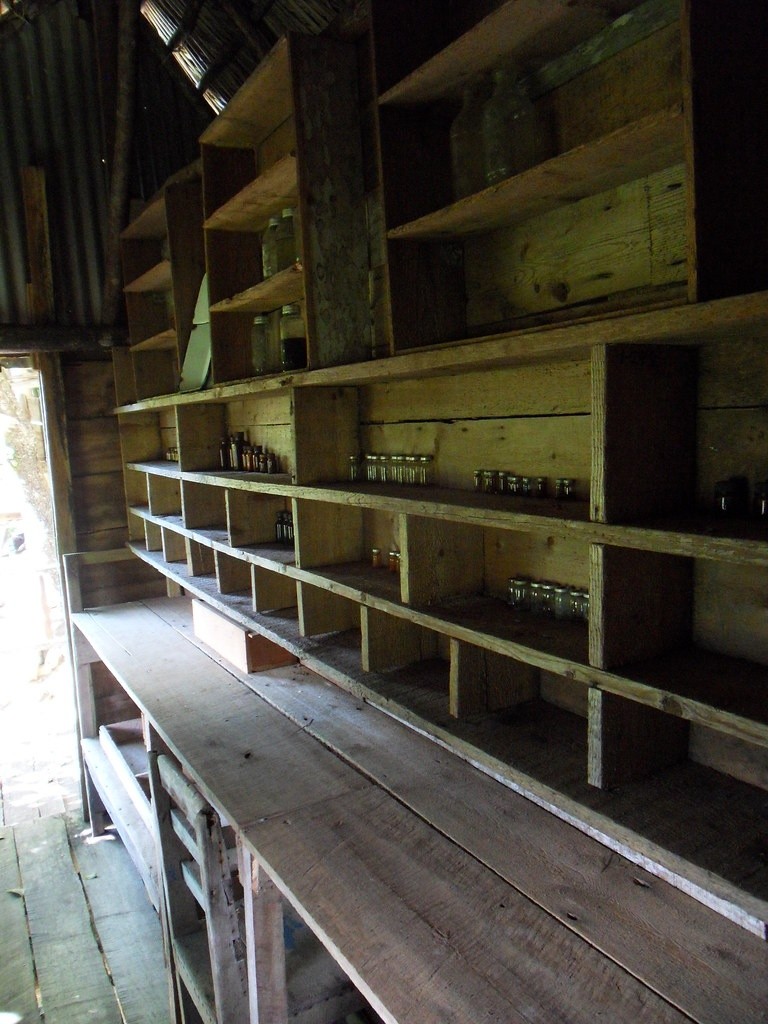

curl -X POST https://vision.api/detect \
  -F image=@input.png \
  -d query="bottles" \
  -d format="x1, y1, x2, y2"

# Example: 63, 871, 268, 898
480, 68, 538, 188
275, 207, 299, 272
250, 316, 270, 377
220, 432, 277, 474
372, 549, 400, 572
473, 470, 574, 500
348, 456, 360, 482
262, 218, 278, 279
713, 480, 737, 519
275, 511, 294, 546
279, 304, 307, 371
753, 484, 768, 523
267, 309, 283, 374
448, 78, 485, 202
366, 455, 434, 487
165, 447, 178, 462
507, 578, 590, 620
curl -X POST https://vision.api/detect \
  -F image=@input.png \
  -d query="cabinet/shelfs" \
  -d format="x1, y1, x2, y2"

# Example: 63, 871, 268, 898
103, 0, 768, 936
70, 618, 395, 1024
